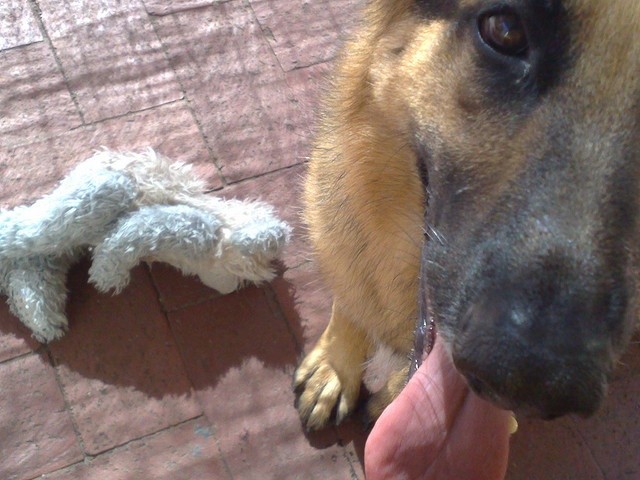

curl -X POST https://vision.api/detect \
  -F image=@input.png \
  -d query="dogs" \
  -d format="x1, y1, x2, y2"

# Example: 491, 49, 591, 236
292, 1, 640, 480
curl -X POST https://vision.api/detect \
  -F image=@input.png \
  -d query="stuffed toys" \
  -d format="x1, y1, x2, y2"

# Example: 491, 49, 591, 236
1, 146, 294, 346
